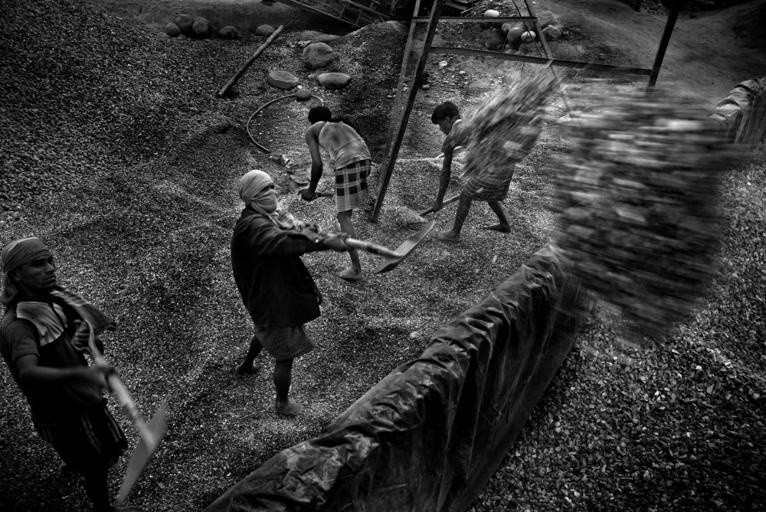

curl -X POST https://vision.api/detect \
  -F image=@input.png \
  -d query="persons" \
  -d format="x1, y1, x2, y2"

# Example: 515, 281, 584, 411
298, 105, 374, 283
231, 168, 362, 418
0, 236, 130, 509
427, 101, 512, 244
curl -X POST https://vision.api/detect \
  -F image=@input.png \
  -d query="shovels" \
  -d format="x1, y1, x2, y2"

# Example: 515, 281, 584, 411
400, 194, 462, 225
299, 220, 436, 274
70, 318, 168, 505
